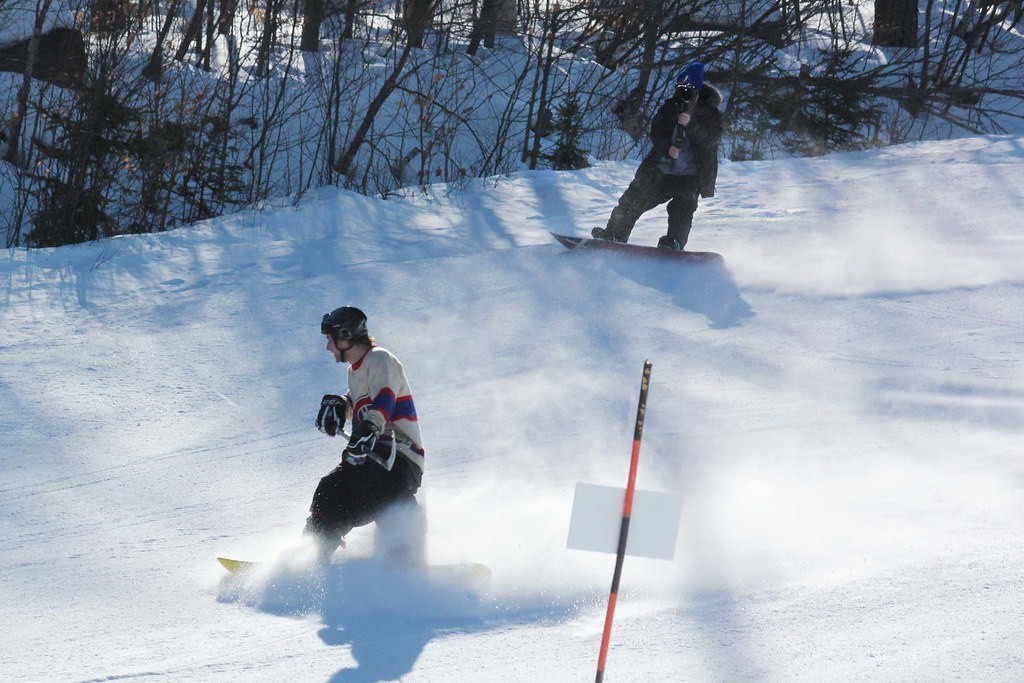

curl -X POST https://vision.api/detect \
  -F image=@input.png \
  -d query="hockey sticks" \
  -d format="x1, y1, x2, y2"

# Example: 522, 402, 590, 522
334, 428, 400, 472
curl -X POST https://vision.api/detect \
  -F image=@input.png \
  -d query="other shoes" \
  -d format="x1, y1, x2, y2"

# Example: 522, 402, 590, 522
592, 227, 626, 243
658, 236, 681, 250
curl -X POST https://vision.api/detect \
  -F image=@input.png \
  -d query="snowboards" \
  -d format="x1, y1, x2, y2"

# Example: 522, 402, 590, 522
548, 229, 725, 267
217, 555, 490, 597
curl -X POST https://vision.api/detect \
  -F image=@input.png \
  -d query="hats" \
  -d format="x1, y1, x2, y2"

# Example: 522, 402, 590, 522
677, 62, 704, 90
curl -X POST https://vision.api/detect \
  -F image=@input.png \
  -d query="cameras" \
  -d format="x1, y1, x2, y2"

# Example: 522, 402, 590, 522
676, 84, 697, 102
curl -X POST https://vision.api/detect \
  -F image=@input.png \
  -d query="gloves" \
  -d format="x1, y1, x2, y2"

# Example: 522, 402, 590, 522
342, 427, 377, 465
315, 395, 346, 437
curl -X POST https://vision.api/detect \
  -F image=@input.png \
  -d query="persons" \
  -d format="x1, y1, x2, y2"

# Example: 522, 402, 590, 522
592, 63, 723, 251
303, 305, 425, 557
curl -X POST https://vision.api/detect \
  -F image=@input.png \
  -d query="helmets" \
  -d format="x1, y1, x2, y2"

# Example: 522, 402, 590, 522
321, 307, 367, 340
